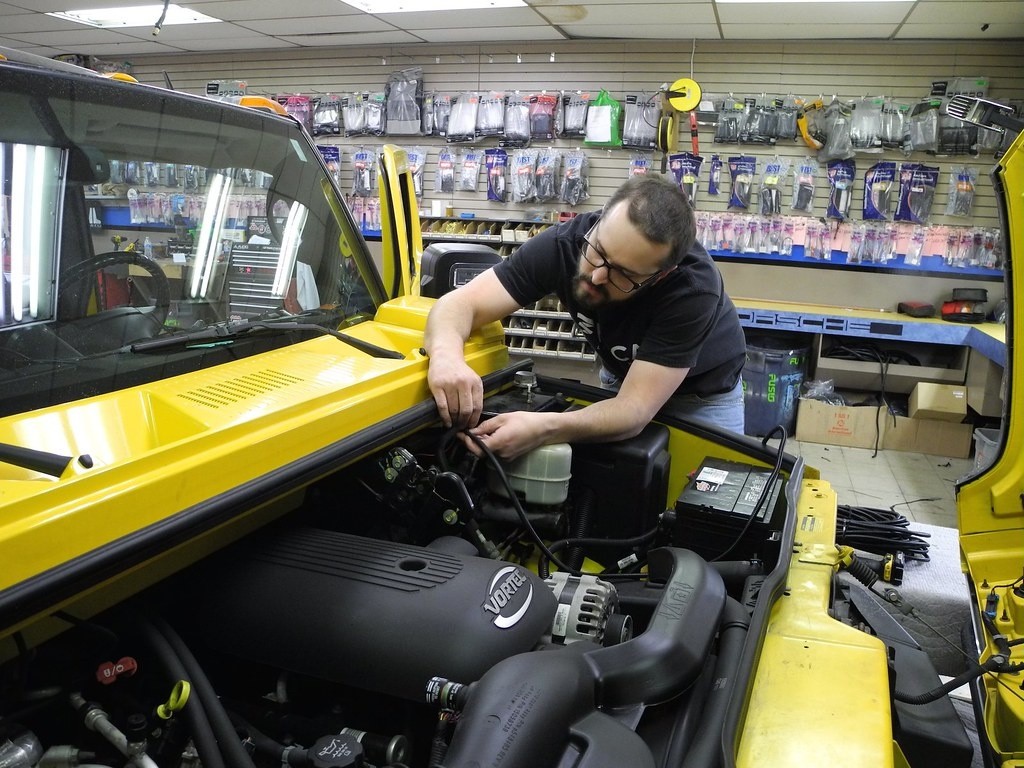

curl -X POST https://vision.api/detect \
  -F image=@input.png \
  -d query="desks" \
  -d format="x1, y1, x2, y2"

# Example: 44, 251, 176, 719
127, 258, 227, 305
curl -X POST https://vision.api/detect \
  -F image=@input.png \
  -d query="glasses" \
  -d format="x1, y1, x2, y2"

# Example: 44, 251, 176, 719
581, 214, 665, 294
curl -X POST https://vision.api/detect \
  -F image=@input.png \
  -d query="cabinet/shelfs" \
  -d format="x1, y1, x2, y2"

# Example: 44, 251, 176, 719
420, 216, 598, 362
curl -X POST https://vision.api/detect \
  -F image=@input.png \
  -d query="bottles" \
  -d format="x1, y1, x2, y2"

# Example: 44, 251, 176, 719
144, 236, 152, 259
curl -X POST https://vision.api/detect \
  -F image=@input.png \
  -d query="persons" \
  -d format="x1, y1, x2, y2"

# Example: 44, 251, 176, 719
423, 172, 748, 459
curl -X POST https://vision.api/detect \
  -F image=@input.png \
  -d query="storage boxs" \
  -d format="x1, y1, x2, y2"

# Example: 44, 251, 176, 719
794, 392, 888, 450
972, 428, 1000, 471
420, 220, 598, 360
247, 216, 288, 246
222, 229, 247, 242
883, 413, 973, 459
908, 381, 968, 422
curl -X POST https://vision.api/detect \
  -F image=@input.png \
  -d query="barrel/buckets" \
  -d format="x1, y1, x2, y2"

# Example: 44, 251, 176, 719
741, 335, 812, 439
